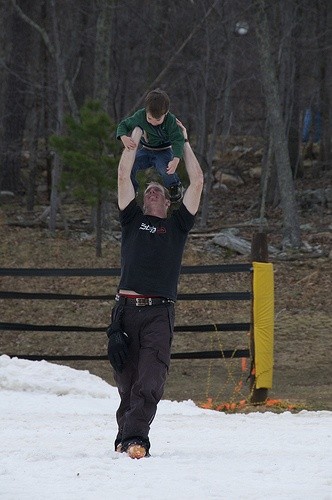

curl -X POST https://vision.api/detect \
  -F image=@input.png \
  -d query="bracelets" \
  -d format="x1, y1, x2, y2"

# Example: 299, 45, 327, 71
184, 139, 189, 142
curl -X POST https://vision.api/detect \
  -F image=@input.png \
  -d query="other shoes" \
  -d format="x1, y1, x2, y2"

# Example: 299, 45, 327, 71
134, 190, 140, 207
168, 181, 185, 202
126, 444, 146, 459
116, 442, 122, 452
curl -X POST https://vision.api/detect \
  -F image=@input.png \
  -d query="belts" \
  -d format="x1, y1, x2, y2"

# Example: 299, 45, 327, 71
114, 295, 174, 307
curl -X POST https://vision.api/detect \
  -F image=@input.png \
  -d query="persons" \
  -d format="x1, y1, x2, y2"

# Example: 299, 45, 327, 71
105, 115, 204, 459
116, 89, 185, 205
